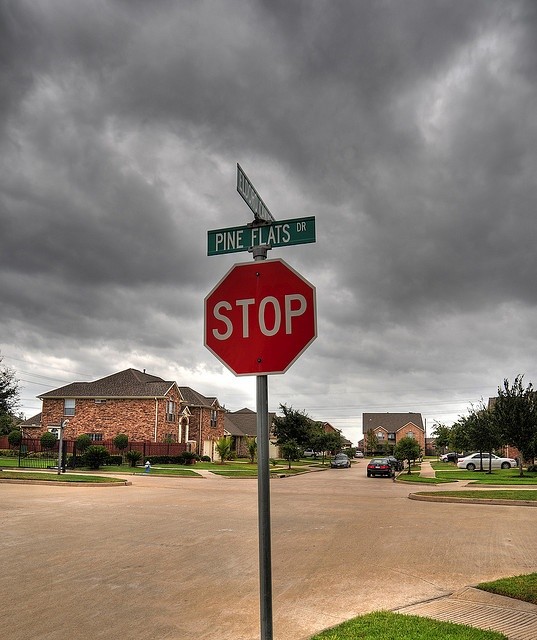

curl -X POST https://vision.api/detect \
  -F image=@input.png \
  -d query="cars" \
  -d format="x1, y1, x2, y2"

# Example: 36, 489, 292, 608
355, 451, 363, 458
446, 451, 476, 463
456, 452, 517, 470
330, 454, 351, 468
439, 452, 463, 462
387, 456, 404, 472
366, 458, 395, 478
301, 449, 318, 458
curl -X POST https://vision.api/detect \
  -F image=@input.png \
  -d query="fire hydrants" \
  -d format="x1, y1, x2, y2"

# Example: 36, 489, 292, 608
145, 461, 151, 473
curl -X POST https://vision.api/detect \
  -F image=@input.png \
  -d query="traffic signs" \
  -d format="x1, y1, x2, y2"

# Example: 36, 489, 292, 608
236, 162, 279, 222
208, 214, 316, 256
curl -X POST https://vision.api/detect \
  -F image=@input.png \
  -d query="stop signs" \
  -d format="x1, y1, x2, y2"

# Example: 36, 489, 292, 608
203, 259, 317, 376
